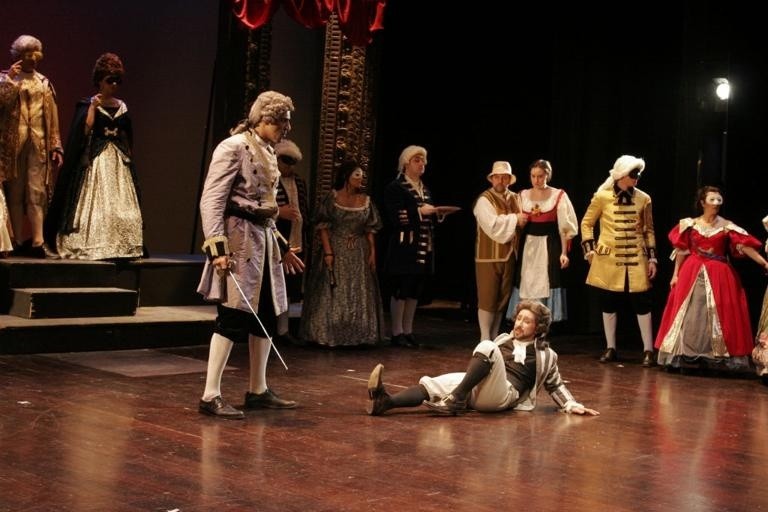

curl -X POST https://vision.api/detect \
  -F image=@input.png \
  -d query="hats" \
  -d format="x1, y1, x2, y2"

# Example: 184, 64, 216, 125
486, 160, 516, 186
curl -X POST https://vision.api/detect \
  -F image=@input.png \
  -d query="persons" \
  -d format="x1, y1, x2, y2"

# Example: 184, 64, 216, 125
664, 182, 768, 376
472, 160, 528, 347
40, 53, 147, 259
749, 213, 768, 376
367, 299, 601, 419
513, 157, 579, 306
198, 90, 306, 420
579, 154, 656, 366
377, 144, 460, 348
295, 159, 385, 346
267, 139, 306, 348
0, 35, 62, 257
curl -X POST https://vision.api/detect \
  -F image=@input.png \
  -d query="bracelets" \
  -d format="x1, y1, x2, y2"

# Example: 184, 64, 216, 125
324, 253, 333, 256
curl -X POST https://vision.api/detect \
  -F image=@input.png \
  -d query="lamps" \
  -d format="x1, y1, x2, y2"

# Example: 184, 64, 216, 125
710, 76, 736, 218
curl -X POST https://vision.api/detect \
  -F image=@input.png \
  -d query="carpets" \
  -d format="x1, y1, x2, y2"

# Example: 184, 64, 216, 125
36, 348, 237, 378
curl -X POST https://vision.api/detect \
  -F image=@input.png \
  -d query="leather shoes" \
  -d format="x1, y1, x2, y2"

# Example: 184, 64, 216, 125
26, 242, 62, 261
364, 362, 391, 415
197, 395, 244, 420
600, 347, 619, 363
390, 333, 424, 347
421, 394, 469, 414
244, 389, 297, 411
639, 349, 654, 367
